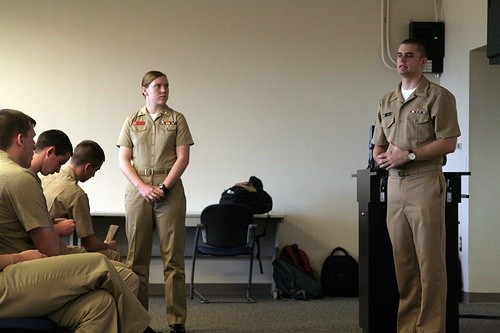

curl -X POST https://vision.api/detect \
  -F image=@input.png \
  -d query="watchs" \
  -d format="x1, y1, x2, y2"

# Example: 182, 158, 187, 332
407, 149, 416, 162
159, 184, 169, 194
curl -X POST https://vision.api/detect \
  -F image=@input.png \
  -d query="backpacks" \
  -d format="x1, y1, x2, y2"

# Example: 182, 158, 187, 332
220, 176, 273, 215
320, 247, 362, 297
272, 244, 319, 301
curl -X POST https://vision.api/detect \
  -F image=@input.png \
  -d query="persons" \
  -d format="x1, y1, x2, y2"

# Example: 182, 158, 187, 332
371, 38, 461, 333
0, 109, 154, 332
116, 70, 195, 333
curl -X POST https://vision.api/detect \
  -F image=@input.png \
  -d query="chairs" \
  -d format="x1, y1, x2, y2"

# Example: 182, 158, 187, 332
189, 204, 262, 304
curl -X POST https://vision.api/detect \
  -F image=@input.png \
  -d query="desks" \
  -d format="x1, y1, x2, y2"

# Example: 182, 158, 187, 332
63, 214, 285, 261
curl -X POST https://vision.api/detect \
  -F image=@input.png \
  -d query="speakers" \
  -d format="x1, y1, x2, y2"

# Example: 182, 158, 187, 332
408, 21, 445, 73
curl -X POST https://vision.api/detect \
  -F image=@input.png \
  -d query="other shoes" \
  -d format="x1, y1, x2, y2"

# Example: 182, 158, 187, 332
143, 326, 155, 333
169, 323, 186, 333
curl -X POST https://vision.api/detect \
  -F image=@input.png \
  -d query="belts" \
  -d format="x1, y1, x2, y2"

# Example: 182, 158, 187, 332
388, 164, 442, 177
137, 168, 170, 176
